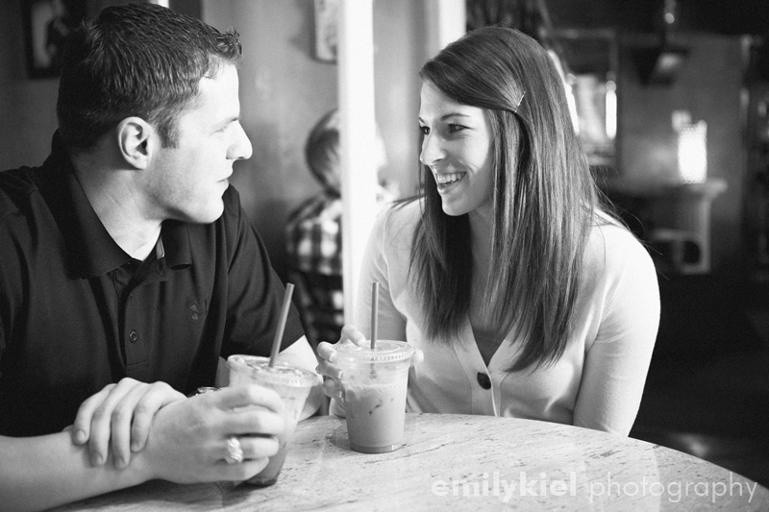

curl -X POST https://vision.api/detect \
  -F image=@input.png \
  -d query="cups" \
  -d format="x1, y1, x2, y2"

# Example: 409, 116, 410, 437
338, 338, 418, 454
225, 355, 324, 486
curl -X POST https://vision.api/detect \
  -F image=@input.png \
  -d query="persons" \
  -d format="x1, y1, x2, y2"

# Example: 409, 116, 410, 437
283, 103, 340, 345
0, 0, 326, 512
314, 22, 662, 437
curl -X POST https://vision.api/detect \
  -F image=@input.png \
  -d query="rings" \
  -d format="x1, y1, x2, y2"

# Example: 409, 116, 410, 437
222, 435, 248, 463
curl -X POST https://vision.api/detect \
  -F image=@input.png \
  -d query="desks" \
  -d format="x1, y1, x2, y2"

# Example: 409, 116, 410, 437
52, 409, 769, 510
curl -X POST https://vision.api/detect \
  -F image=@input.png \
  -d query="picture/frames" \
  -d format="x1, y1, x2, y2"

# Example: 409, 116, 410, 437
22, 1, 87, 80
